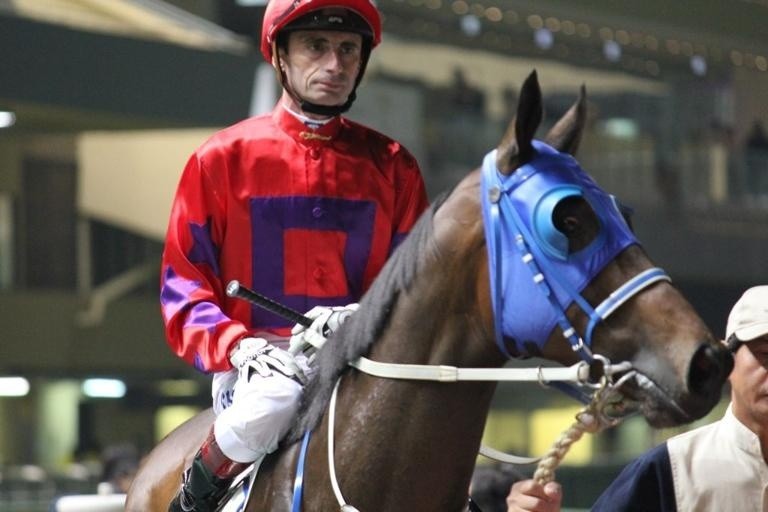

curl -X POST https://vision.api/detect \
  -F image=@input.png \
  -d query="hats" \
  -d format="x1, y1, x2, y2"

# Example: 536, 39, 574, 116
725, 285, 768, 341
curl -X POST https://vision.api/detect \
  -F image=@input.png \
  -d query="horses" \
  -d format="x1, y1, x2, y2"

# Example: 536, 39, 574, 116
125, 67, 735, 512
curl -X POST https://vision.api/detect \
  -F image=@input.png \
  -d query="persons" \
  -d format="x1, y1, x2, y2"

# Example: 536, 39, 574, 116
503, 282, 768, 512
158, 1, 433, 512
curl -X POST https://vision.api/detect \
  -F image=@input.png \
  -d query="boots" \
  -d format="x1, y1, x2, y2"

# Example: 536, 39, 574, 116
168, 450, 234, 512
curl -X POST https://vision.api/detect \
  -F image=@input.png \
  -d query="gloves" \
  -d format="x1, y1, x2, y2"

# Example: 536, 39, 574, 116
230, 303, 360, 385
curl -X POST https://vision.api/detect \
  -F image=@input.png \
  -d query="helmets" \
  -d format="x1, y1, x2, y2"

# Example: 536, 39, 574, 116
261, 0, 381, 64
